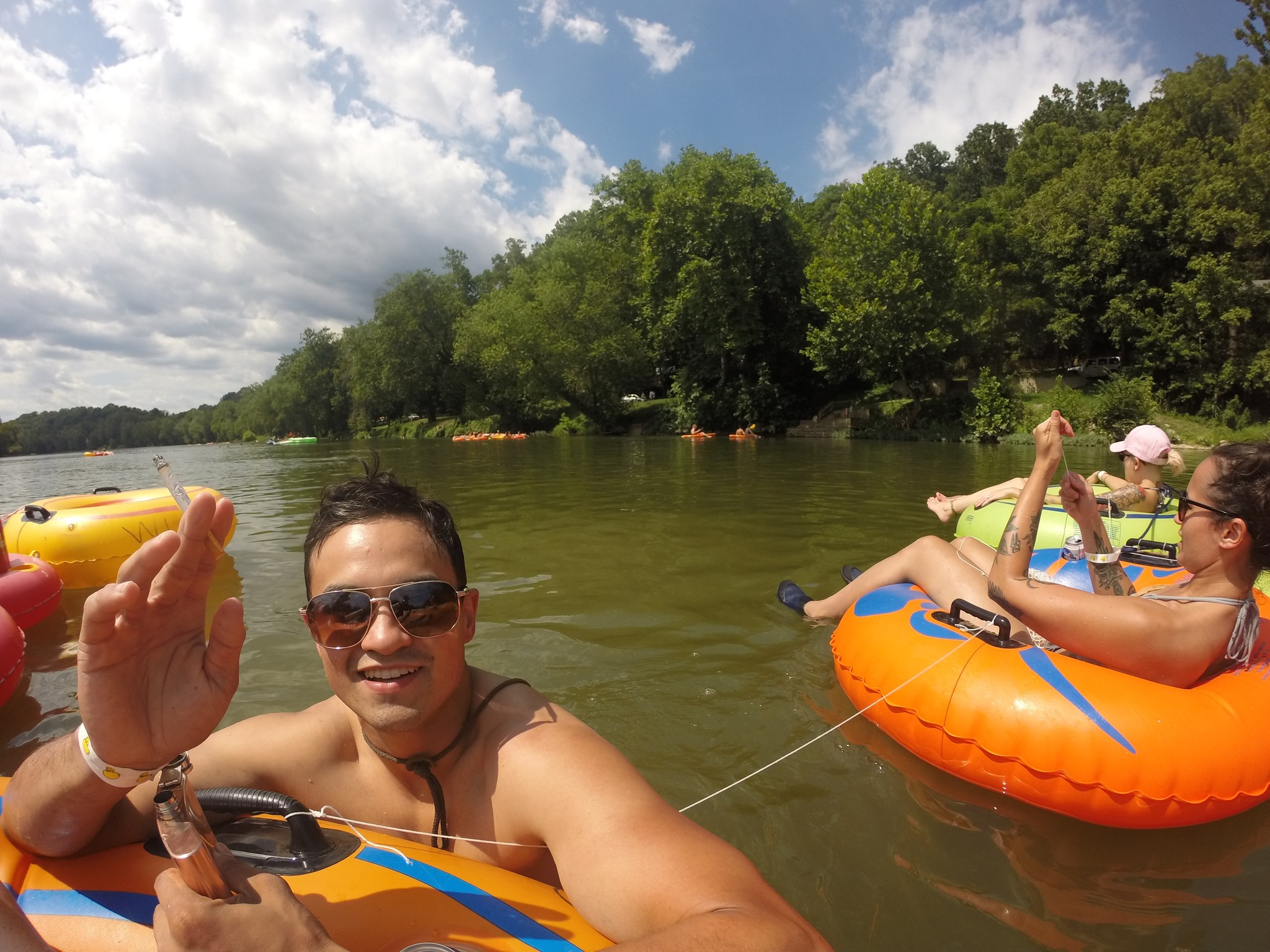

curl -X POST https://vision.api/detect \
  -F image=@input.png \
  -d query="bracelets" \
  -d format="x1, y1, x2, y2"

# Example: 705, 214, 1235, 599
1083, 549, 1121, 567
78, 719, 164, 789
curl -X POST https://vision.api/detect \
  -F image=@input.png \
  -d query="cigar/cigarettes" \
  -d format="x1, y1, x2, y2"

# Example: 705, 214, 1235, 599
149, 454, 228, 561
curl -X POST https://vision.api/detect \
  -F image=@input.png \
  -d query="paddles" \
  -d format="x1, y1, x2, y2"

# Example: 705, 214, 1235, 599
745, 423, 756, 432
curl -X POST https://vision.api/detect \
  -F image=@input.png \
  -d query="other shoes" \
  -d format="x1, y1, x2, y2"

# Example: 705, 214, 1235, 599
776, 580, 813, 618
840, 564, 862, 584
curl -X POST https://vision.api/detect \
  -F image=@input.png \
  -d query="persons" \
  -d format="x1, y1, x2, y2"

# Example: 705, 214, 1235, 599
735, 424, 758, 439
689, 423, 713, 438
0, 449, 835, 952
452, 430, 526, 441
774, 409, 1270, 690
925, 422, 1186, 527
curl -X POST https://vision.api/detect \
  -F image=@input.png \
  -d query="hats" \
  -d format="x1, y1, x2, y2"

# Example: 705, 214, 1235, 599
1110, 425, 1171, 465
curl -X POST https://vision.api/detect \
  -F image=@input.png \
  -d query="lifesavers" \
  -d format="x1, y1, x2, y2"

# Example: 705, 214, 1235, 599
0, 772, 618, 952
954, 484, 1186, 557
95, 451, 113, 456
0, 605, 27, 707
454, 434, 527, 441
827, 548, 1270, 829
2, 484, 239, 592
84, 451, 96, 457
0, 553, 64, 630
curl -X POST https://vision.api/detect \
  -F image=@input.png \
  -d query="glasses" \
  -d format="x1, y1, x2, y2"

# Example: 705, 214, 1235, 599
1119, 452, 1146, 465
297, 579, 469, 650
1177, 490, 1258, 541
